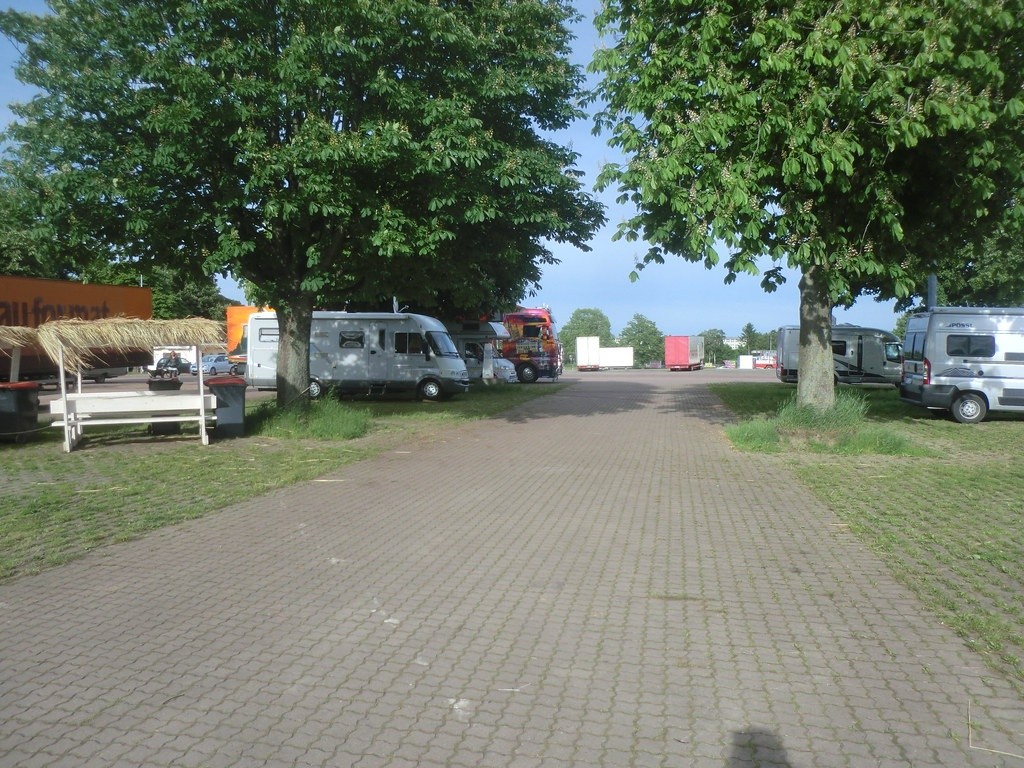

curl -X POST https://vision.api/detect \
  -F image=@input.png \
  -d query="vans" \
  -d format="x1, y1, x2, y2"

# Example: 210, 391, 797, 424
244, 311, 470, 402
776, 325, 903, 387
156, 358, 191, 373
900, 307, 1024, 424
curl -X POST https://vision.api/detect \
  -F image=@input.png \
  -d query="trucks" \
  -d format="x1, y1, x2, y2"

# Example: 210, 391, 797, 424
665, 336, 704, 371
453, 339, 517, 382
190, 353, 238, 376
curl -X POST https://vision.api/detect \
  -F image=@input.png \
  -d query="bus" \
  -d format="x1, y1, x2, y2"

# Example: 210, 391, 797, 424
751, 349, 776, 369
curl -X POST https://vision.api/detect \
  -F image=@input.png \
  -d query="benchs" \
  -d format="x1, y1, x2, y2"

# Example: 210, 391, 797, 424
51, 414, 218, 427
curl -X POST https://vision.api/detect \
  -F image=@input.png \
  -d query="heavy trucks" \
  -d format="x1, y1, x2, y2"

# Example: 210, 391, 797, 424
481, 308, 563, 383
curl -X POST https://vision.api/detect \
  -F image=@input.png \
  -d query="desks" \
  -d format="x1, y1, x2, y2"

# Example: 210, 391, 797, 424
49, 391, 218, 454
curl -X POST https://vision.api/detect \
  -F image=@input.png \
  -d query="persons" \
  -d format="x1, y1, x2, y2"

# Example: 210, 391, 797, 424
163, 351, 180, 378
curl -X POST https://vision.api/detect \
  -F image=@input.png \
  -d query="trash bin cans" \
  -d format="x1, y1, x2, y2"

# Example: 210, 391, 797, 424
0, 382, 40, 445
204, 376, 249, 438
146, 379, 183, 436
